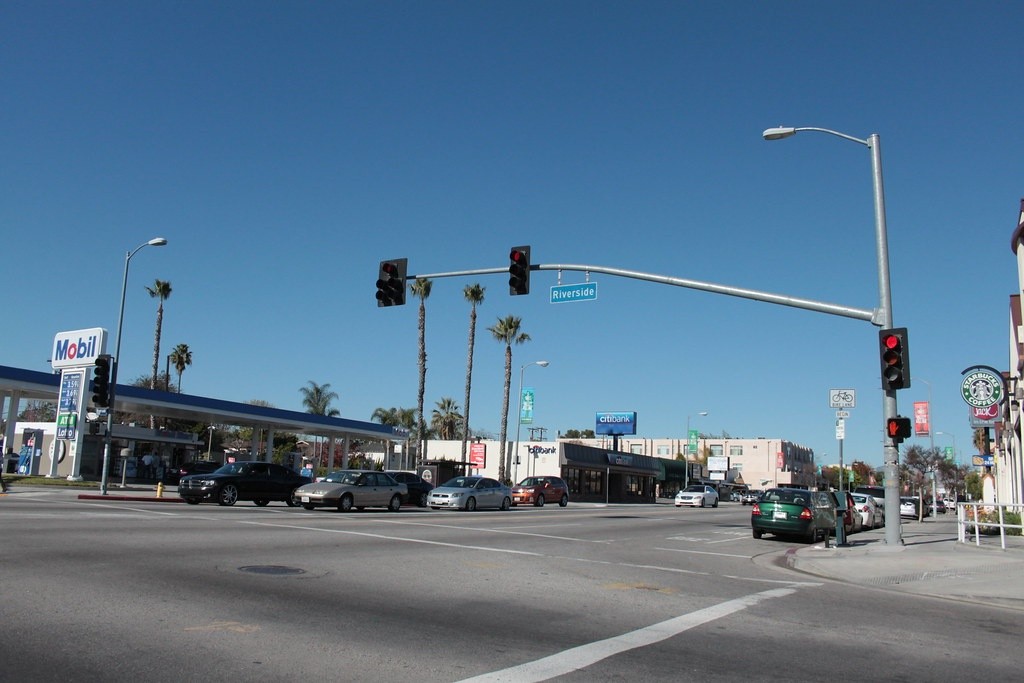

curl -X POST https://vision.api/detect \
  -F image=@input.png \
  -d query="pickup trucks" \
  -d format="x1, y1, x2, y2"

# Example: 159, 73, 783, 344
943, 497, 955, 510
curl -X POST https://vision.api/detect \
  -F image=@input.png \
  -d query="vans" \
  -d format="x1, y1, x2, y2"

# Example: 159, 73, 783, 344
852, 486, 885, 508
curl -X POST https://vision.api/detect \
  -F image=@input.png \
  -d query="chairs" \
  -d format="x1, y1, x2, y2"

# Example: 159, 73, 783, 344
794, 497, 804, 505
770, 495, 779, 501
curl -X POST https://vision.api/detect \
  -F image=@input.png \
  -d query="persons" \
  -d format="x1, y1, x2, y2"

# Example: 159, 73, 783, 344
141, 452, 161, 480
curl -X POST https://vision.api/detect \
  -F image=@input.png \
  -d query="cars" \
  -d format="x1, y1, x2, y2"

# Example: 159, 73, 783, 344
730, 492, 766, 504
384, 471, 433, 508
294, 469, 408, 512
511, 475, 569, 507
751, 487, 840, 544
675, 485, 720, 508
850, 493, 885, 530
426, 476, 513, 511
929, 500, 946, 514
832, 491, 863, 533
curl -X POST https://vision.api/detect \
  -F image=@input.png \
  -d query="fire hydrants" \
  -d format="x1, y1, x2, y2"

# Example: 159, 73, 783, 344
156, 481, 163, 498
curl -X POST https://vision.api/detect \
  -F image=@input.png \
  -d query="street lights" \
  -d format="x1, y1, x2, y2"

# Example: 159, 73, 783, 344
685, 412, 707, 487
207, 425, 215, 461
100, 238, 171, 493
936, 432, 958, 514
513, 361, 549, 484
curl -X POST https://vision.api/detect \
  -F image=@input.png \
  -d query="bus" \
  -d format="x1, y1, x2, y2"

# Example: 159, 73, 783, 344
177, 461, 314, 506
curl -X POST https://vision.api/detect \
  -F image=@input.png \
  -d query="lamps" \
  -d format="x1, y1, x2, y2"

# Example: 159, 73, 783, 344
999, 443, 1005, 451
1003, 430, 1013, 438
1011, 400, 1020, 411
1015, 388, 1024, 399
1006, 422, 1015, 431
999, 451, 1004, 457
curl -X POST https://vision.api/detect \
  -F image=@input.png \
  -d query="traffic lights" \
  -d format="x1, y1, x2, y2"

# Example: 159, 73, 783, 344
509, 245, 530, 295
375, 257, 408, 307
879, 327, 911, 389
92, 354, 111, 407
887, 418, 912, 437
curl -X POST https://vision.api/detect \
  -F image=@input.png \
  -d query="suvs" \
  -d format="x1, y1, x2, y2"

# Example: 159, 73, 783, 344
176, 461, 222, 485
899, 496, 930, 519
